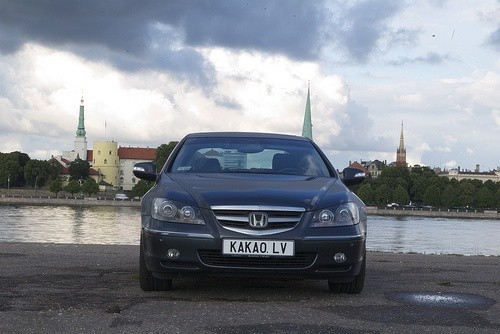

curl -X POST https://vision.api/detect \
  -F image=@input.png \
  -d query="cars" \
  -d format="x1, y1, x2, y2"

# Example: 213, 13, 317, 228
134, 130, 368, 295
114, 193, 129, 202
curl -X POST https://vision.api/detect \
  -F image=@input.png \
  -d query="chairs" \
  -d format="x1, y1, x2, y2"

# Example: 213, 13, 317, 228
189, 153, 310, 173
